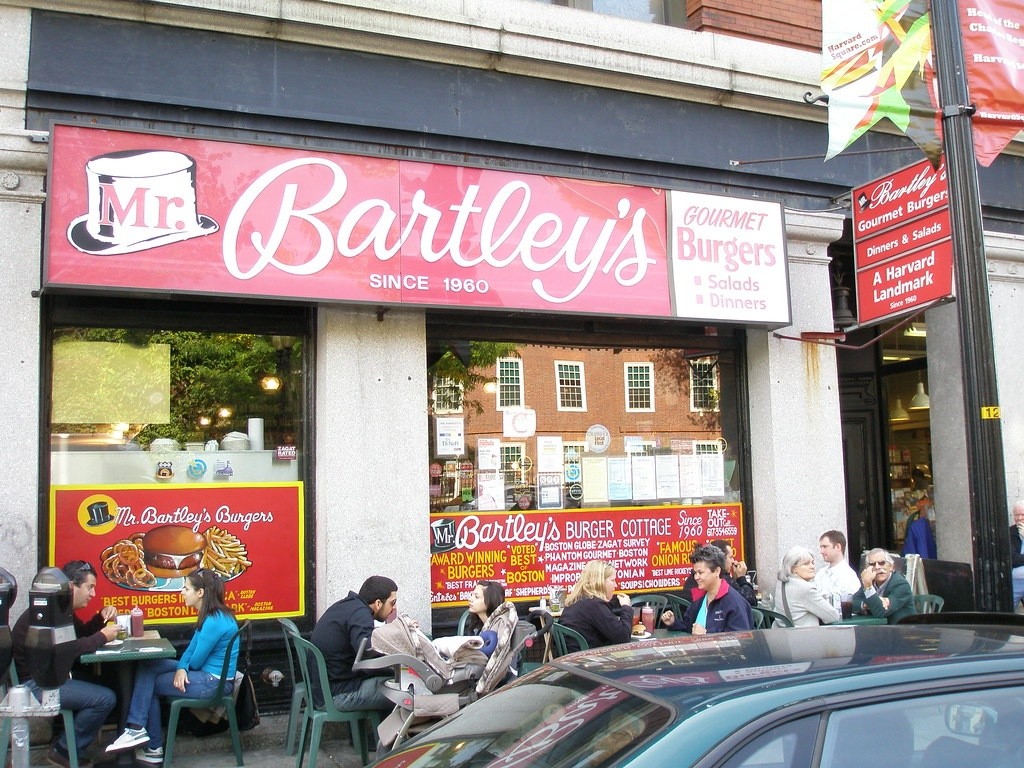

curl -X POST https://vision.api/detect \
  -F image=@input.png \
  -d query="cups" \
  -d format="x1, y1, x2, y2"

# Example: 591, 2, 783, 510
753, 585, 759, 596
840, 595, 853, 619
633, 607, 640, 625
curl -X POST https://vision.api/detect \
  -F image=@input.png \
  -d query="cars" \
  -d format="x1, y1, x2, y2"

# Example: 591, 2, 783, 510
358, 611, 1024, 768
444, 485, 572, 513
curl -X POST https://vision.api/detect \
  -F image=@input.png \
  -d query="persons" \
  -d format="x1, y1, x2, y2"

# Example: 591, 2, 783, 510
106, 569, 240, 768
13, 562, 120, 768
814, 530, 861, 602
469, 580, 505, 633
307, 576, 419, 757
554, 562, 634, 657
852, 549, 915, 625
1009, 502, 1024, 608
771, 548, 840, 627
510, 497, 536, 510
680, 540, 758, 616
662, 546, 754, 634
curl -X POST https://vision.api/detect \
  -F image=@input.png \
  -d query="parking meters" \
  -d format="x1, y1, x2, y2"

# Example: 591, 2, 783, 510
0, 567, 76, 768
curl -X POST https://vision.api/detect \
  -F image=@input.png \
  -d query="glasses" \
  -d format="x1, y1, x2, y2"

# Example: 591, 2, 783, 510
868, 560, 888, 566
197, 568, 205, 581
69, 561, 93, 580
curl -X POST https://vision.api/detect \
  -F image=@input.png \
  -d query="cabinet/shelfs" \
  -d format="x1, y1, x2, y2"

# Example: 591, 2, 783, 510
890, 460, 913, 488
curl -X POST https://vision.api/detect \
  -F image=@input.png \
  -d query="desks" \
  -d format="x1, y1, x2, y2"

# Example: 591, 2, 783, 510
630, 629, 693, 642
831, 613, 888, 625
529, 606, 566, 663
80, 629, 177, 768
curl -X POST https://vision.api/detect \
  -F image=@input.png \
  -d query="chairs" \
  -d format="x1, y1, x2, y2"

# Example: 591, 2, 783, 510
550, 621, 589, 656
912, 594, 945, 613
665, 593, 692, 620
277, 616, 383, 768
832, 708, 916, 768
630, 596, 668, 628
748, 605, 793, 629
163, 619, 252, 768
0, 658, 80, 768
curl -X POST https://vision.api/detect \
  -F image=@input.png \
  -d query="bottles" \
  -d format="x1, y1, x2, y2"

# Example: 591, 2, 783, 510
641, 607, 654, 635
118, 626, 128, 639
549, 598, 560, 611
131, 607, 143, 636
540, 596, 546, 611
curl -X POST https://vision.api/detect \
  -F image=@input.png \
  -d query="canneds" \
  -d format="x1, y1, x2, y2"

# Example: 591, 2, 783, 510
822, 593, 833, 607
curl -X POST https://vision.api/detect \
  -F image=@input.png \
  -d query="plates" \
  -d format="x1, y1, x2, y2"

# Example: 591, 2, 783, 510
104, 640, 124, 648
631, 632, 651, 640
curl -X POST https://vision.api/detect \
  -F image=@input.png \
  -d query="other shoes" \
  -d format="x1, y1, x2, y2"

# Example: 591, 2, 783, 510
47, 736, 94, 768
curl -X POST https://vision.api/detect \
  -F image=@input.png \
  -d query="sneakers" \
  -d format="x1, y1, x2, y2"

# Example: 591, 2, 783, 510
105, 726, 151, 753
134, 746, 164, 763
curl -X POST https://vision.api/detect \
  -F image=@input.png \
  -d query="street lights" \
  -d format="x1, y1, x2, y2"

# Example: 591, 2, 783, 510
270, 334, 297, 446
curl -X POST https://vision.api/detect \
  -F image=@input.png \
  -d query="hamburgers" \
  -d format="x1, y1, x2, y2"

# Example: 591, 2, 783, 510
632, 625, 646, 635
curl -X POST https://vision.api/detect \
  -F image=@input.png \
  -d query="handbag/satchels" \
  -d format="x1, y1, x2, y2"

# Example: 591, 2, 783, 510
180, 704, 230, 738
234, 671, 260, 731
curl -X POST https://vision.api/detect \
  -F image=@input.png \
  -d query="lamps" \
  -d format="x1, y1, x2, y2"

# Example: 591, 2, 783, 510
888, 320, 910, 421
904, 322, 927, 338
909, 370, 930, 409
